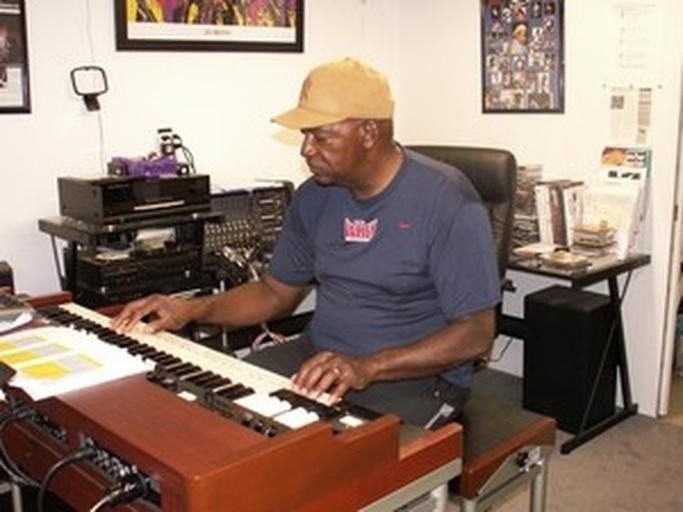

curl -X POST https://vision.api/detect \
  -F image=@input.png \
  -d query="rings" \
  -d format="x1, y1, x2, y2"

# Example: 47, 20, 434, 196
330, 367, 341, 378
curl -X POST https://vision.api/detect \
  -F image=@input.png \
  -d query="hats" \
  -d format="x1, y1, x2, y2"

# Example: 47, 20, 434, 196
270, 55, 395, 131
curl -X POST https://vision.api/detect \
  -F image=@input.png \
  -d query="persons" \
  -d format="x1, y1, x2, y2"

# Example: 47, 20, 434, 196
108, 61, 500, 430
482, 0, 558, 109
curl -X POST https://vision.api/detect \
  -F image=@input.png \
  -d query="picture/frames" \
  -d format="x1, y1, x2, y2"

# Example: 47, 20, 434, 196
0, 1, 30, 113
482, 1, 564, 113
115, 0, 304, 53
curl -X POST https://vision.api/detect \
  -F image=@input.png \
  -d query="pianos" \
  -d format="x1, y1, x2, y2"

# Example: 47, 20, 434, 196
29, 290, 385, 438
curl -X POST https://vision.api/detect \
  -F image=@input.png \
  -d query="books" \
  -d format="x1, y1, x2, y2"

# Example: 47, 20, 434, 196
516, 140, 651, 268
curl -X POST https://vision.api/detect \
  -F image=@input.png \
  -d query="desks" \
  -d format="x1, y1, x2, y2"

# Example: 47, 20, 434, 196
500, 246, 651, 454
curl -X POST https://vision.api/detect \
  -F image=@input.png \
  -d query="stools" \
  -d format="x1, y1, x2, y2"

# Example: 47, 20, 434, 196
449, 395, 556, 512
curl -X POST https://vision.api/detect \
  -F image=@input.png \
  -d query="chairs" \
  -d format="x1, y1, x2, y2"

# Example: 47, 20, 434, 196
407, 146, 515, 370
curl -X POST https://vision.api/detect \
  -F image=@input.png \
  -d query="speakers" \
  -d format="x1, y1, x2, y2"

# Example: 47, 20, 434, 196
523, 283, 619, 436
